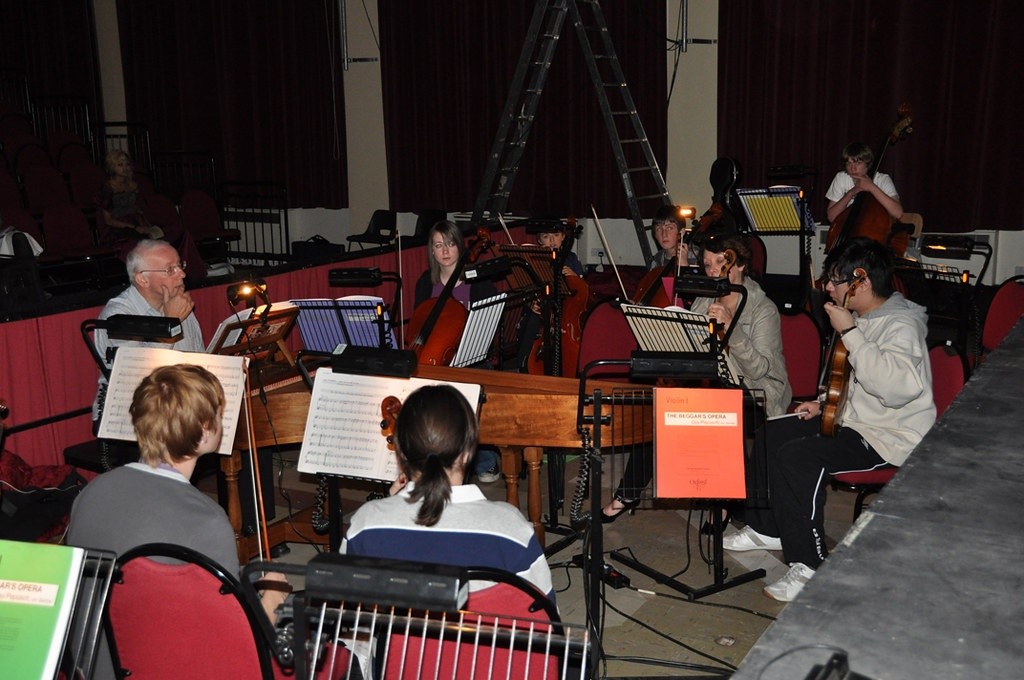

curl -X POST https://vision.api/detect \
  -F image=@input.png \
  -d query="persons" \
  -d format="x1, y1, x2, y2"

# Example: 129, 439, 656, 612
100, 148, 207, 282
629, 204, 701, 305
515, 232, 584, 351
825, 141, 903, 223
414, 219, 502, 482
599, 233, 793, 535
92, 238, 291, 559
337, 384, 560, 617
0, 449, 88, 543
67, 364, 291, 680
722, 236, 938, 602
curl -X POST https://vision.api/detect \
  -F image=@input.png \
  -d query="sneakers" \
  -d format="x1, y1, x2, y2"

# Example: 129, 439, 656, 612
723, 525, 785, 551
762, 562, 816, 601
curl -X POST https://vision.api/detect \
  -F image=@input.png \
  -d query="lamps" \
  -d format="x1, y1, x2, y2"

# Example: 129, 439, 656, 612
574, 349, 719, 680
526, 218, 584, 377
240, 551, 469, 666
765, 164, 821, 280
297, 343, 417, 552
81, 314, 183, 382
673, 206, 699, 230
463, 254, 578, 559
922, 235, 993, 353
328, 268, 402, 348
227, 280, 273, 323
610, 275, 766, 602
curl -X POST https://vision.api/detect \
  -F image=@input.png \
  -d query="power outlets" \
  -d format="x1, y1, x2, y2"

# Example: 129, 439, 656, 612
590, 247, 610, 257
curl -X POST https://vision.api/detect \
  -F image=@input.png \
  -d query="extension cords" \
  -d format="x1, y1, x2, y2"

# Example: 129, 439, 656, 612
572, 555, 630, 589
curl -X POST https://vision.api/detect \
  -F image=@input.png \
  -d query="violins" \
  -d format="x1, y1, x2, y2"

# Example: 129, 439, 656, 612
819, 271, 869, 436
379, 397, 416, 483
673, 249, 737, 391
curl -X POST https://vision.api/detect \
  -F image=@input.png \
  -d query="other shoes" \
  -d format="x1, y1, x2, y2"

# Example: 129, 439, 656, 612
243, 520, 291, 558
477, 461, 500, 483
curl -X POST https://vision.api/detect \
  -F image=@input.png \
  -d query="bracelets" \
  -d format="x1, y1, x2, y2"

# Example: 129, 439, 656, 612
840, 325, 857, 337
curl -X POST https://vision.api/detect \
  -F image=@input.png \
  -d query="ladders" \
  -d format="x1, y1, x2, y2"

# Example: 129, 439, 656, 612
470, 0, 672, 272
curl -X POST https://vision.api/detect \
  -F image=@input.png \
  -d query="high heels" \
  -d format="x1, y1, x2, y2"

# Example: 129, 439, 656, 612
700, 508, 733, 535
600, 495, 641, 524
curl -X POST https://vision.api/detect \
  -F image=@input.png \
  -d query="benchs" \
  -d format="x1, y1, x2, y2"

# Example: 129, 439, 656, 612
63, 440, 220, 482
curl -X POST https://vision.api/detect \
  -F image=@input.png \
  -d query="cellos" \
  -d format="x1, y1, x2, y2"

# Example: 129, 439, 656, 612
631, 201, 722, 308
403, 226, 492, 365
822, 92, 914, 265
518, 214, 588, 377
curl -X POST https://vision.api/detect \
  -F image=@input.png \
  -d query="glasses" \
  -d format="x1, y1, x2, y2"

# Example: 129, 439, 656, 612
823, 275, 855, 286
139, 260, 187, 277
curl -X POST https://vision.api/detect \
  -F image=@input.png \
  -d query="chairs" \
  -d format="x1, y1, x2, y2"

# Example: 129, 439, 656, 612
0, 101, 1024, 680
